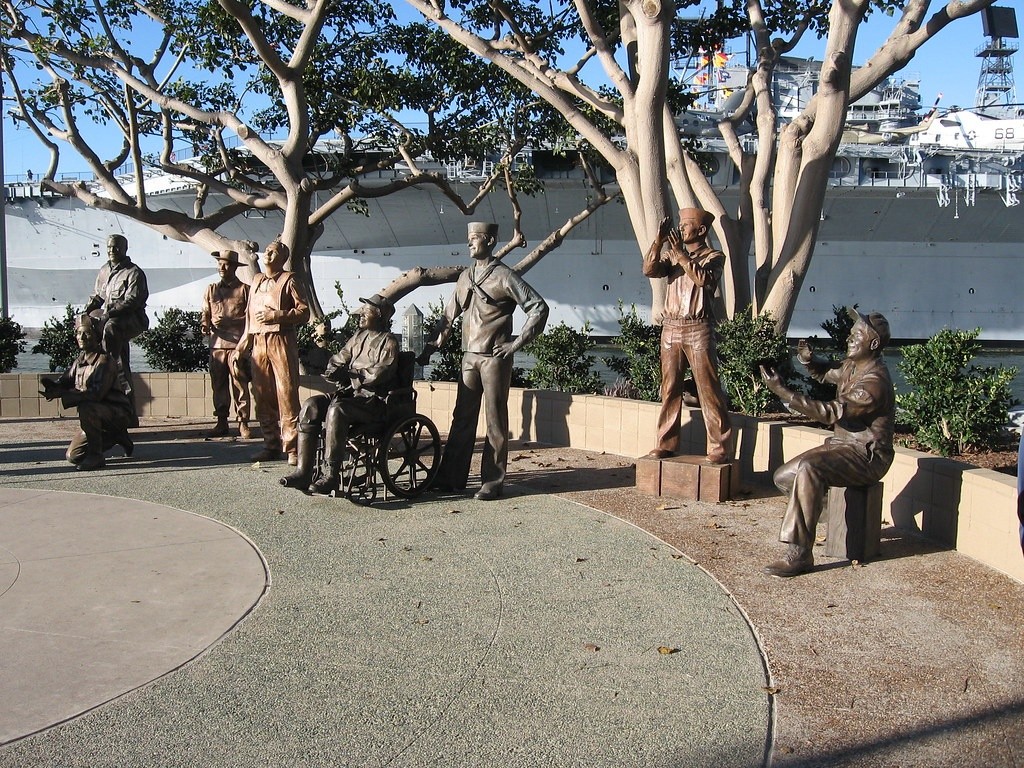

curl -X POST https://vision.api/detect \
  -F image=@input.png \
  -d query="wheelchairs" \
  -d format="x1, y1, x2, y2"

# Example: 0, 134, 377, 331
312, 351, 444, 501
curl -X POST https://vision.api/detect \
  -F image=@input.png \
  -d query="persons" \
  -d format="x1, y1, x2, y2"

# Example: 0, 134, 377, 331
643, 208, 735, 465
37, 222, 549, 500
759, 304, 895, 577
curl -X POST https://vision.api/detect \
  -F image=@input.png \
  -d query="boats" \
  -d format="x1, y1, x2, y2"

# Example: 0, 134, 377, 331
665, 16, 1024, 189
